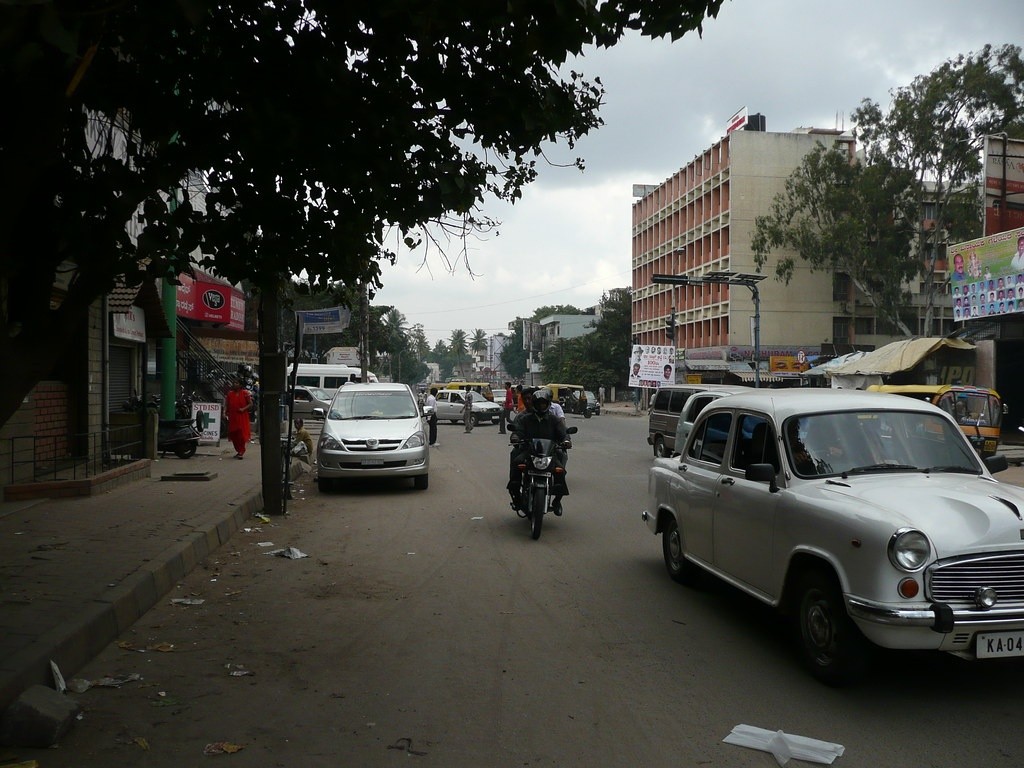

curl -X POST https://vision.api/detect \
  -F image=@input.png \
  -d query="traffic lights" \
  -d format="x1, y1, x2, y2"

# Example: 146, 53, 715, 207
665, 318, 676, 341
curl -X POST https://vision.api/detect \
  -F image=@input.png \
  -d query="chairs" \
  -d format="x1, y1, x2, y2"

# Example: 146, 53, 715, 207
739, 420, 782, 473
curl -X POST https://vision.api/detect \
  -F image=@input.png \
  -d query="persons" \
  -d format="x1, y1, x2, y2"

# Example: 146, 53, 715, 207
223, 378, 254, 460
296, 392, 305, 400
460, 385, 474, 433
951, 237, 1024, 321
498, 382, 513, 434
516, 385, 525, 413
426, 388, 440, 447
290, 418, 313, 457
631, 346, 674, 387
418, 388, 427, 404
598, 384, 605, 405
506, 387, 573, 516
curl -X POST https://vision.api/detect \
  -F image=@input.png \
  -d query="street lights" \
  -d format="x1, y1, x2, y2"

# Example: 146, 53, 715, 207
672, 247, 686, 345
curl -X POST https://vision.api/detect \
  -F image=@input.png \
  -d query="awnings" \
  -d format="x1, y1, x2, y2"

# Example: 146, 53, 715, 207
799, 337, 978, 375
685, 359, 781, 382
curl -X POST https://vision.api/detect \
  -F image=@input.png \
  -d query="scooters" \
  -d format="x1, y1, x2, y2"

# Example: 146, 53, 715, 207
122, 385, 207, 460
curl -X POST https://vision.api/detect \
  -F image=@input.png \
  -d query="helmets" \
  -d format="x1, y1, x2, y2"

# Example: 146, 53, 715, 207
531, 389, 551, 413
540, 387, 553, 400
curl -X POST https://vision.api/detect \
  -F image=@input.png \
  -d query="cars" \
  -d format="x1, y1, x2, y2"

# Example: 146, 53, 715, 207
288, 384, 333, 420
491, 390, 507, 409
510, 384, 547, 399
573, 391, 601, 415
640, 388, 1024, 692
672, 387, 772, 460
435, 390, 503, 427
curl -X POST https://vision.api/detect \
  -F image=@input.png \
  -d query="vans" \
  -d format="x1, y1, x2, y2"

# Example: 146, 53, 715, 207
646, 384, 751, 459
287, 362, 379, 399
428, 376, 494, 402
858, 383, 1009, 463
311, 382, 436, 492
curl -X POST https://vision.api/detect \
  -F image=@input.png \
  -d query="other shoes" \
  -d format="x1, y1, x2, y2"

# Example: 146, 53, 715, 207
233, 453, 243, 460
552, 499, 562, 516
498, 431, 506, 434
511, 490, 522, 508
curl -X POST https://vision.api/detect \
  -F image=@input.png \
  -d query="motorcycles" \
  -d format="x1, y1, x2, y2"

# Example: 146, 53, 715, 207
506, 423, 578, 541
546, 383, 592, 418
